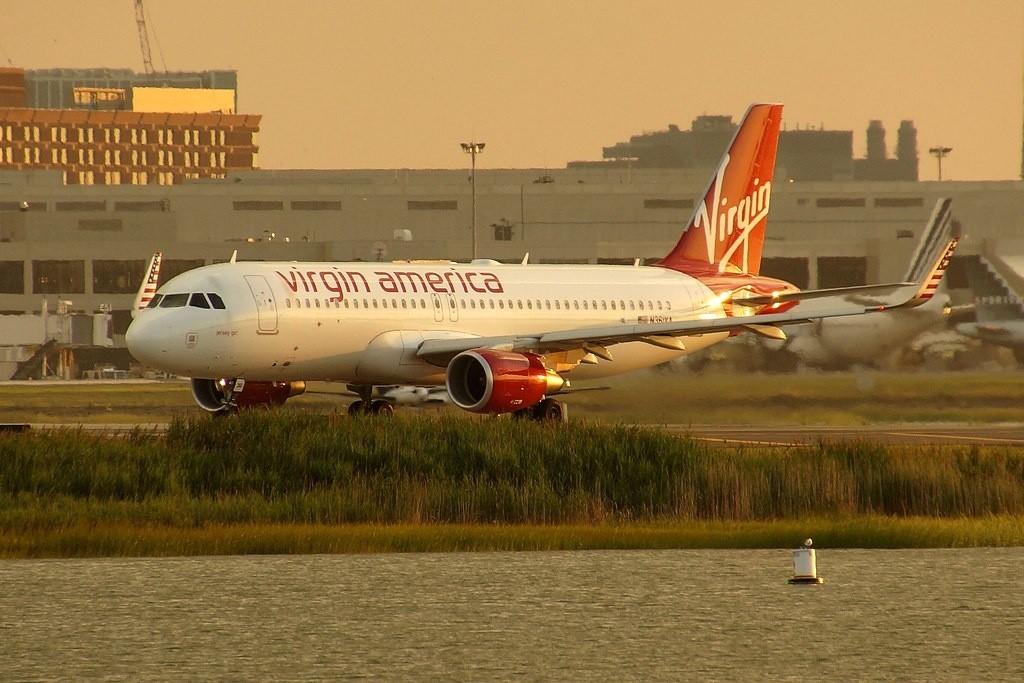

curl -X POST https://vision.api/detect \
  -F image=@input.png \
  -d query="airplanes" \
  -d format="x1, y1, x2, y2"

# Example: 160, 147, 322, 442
683, 273, 980, 378
956, 256, 1024, 367
123, 104, 963, 425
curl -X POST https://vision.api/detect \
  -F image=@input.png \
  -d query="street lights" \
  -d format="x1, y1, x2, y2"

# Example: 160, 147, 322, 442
458, 140, 485, 262
928, 147, 955, 183
